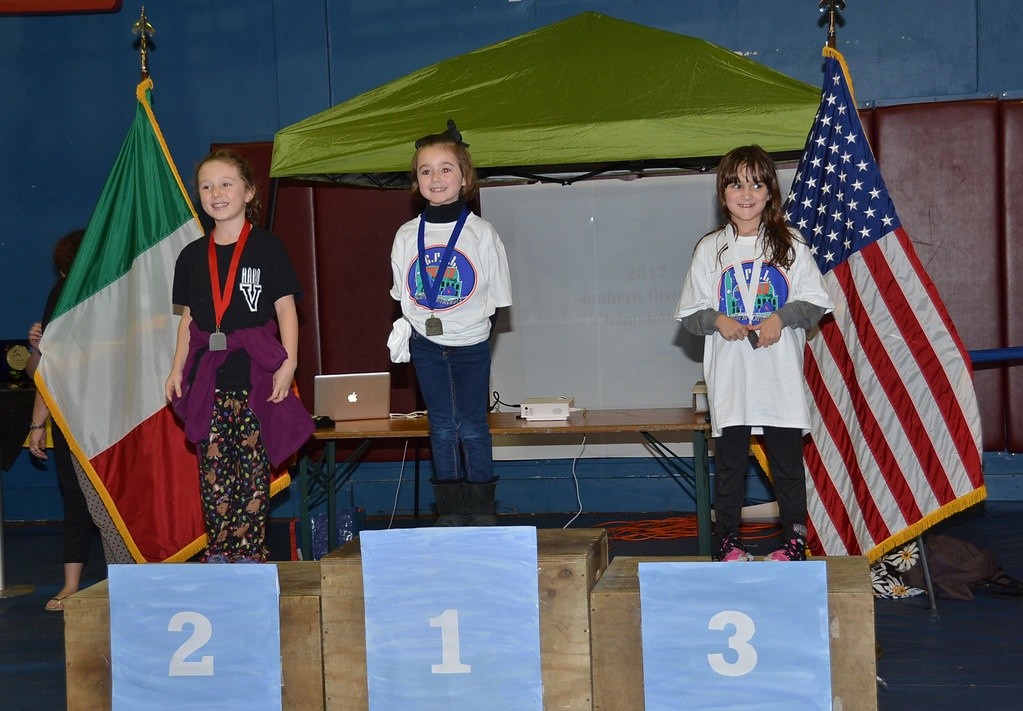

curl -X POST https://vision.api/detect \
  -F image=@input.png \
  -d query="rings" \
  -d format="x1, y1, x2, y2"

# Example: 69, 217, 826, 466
30, 448, 34, 452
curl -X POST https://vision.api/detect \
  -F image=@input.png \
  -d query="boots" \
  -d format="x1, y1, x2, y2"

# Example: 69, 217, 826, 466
427, 477, 466, 526
463, 475, 498, 525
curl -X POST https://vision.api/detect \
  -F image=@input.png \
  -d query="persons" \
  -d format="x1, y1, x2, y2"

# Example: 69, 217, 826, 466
27, 229, 138, 610
390, 120, 513, 528
166, 152, 298, 564
673, 144, 835, 562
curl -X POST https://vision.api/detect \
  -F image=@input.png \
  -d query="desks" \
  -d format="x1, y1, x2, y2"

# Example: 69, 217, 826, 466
297, 407, 714, 556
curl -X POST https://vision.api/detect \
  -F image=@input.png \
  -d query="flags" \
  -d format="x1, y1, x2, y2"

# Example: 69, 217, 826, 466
34, 77, 301, 563
749, 47, 987, 566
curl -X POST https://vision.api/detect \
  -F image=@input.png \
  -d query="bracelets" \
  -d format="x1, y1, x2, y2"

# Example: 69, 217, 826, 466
30, 422, 46, 430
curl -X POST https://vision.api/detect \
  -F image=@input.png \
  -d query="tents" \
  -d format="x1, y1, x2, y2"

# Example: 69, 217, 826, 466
265, 10, 823, 232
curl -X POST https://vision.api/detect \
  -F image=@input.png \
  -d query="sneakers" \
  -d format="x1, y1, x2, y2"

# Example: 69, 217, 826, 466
718, 535, 750, 561
765, 538, 807, 561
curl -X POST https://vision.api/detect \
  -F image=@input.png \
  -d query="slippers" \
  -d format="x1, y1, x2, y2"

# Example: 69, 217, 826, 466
44, 595, 67, 611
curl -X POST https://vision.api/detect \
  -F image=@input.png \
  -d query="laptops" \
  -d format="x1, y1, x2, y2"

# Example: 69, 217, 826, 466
314, 372, 390, 421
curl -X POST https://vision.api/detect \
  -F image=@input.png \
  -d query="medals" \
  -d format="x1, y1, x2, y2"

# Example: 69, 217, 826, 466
747, 330, 760, 350
426, 318, 443, 336
209, 332, 227, 351
